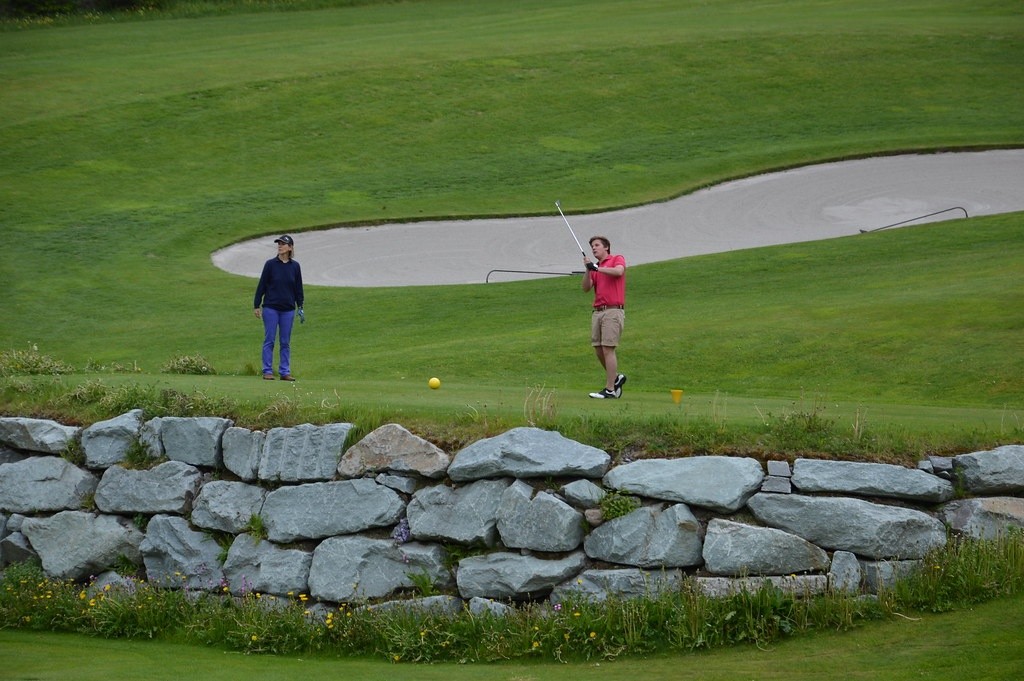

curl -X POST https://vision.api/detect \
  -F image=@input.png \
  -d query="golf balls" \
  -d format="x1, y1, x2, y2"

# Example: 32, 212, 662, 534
428, 376, 441, 389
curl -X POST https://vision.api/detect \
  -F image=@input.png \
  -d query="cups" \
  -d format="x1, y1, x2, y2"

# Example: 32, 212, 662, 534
670, 390, 683, 404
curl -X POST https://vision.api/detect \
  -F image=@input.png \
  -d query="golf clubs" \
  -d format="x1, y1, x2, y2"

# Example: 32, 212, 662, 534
555, 200, 586, 257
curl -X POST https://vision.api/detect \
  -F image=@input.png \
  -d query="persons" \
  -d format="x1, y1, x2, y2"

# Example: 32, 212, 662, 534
254, 235, 304, 382
581, 235, 626, 400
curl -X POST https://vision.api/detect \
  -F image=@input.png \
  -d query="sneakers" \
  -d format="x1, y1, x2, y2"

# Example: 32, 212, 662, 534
589, 388, 618, 400
614, 374, 626, 398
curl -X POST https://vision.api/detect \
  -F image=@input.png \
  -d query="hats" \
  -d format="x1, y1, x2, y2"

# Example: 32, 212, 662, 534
273, 236, 295, 245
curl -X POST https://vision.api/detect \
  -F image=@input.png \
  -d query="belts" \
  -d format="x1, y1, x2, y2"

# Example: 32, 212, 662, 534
594, 305, 624, 310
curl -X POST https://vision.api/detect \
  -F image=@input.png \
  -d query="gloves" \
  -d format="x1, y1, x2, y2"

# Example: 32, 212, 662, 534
297, 309, 305, 323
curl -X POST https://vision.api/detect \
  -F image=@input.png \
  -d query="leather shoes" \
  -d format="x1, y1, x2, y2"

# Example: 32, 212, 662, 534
263, 374, 275, 380
281, 375, 296, 381
